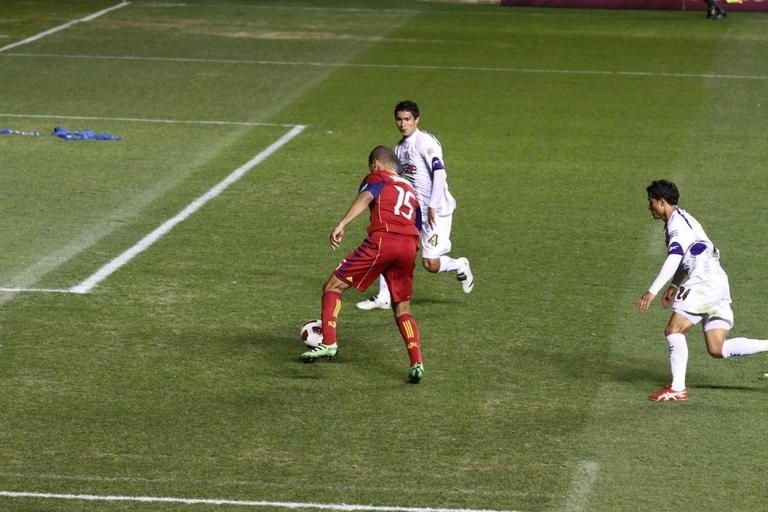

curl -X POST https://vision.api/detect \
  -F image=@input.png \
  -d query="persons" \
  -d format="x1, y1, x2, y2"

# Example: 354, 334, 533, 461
292, 144, 425, 381
353, 97, 476, 311
637, 179, 768, 405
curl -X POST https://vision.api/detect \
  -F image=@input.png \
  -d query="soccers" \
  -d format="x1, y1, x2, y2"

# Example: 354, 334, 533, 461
300, 320, 324, 347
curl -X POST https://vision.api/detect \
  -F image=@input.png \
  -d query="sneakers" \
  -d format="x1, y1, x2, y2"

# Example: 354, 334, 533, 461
297, 342, 340, 364
455, 255, 475, 295
354, 295, 392, 312
405, 361, 424, 382
646, 383, 688, 403
